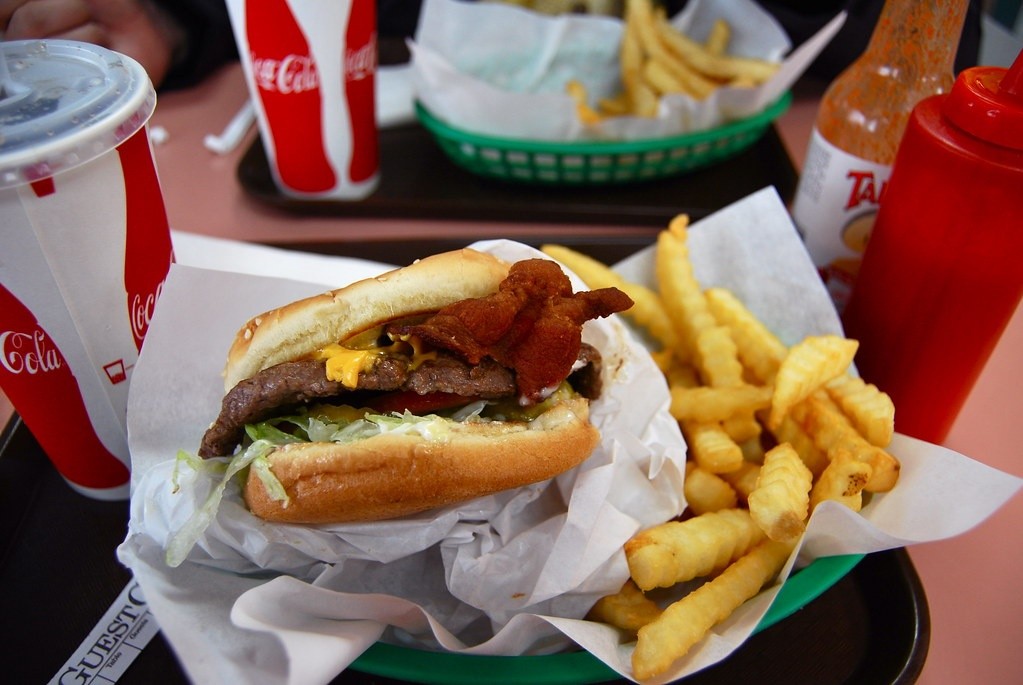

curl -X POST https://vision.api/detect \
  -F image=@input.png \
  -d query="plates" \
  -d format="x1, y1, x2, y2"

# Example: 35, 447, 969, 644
267, 540, 929, 685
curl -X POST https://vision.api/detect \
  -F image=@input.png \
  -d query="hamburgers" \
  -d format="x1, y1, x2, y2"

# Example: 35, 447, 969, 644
168, 248, 638, 566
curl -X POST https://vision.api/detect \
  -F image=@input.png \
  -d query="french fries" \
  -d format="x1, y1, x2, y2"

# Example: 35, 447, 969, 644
537, 210, 900, 683
561, 0, 775, 127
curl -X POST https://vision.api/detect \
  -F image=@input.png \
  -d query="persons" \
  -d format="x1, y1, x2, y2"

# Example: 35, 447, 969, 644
1, 0, 983, 102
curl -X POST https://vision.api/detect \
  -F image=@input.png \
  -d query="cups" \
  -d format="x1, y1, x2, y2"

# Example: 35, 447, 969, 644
0, 36, 185, 502
225, 1, 380, 205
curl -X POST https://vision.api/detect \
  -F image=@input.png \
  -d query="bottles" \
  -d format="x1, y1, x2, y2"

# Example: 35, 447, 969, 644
843, 49, 1023, 462
786, 1, 972, 315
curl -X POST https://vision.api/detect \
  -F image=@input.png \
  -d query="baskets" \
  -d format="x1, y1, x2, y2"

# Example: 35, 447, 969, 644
410, 82, 795, 186
